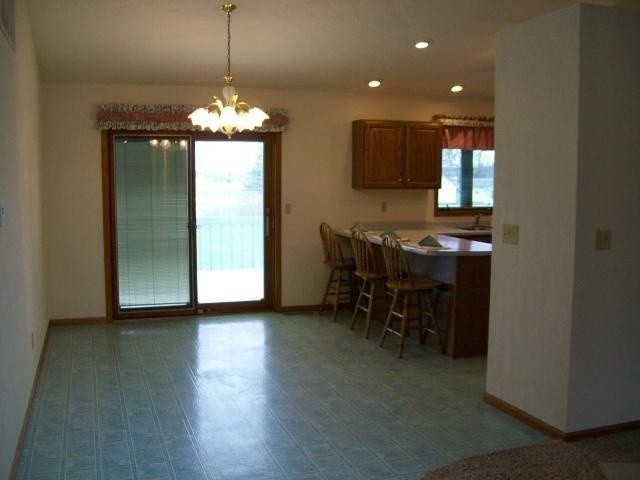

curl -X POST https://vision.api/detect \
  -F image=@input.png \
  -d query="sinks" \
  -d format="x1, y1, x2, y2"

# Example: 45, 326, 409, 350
457, 225, 493, 231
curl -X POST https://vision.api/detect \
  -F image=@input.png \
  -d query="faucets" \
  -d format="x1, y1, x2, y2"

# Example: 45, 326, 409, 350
472, 211, 480, 225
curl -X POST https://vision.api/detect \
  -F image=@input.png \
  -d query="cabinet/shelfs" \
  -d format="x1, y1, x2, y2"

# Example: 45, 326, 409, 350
353, 115, 445, 192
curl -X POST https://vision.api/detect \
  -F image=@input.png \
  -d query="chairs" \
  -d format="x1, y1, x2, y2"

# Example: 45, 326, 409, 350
318, 223, 446, 361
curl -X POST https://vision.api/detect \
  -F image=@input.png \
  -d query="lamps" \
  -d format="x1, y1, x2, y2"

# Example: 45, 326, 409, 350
183, 2, 272, 140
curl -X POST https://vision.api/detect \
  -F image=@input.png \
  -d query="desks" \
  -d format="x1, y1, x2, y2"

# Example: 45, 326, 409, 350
337, 228, 492, 361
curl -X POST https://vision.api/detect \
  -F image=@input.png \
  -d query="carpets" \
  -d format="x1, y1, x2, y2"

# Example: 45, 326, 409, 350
417, 426, 640, 480
